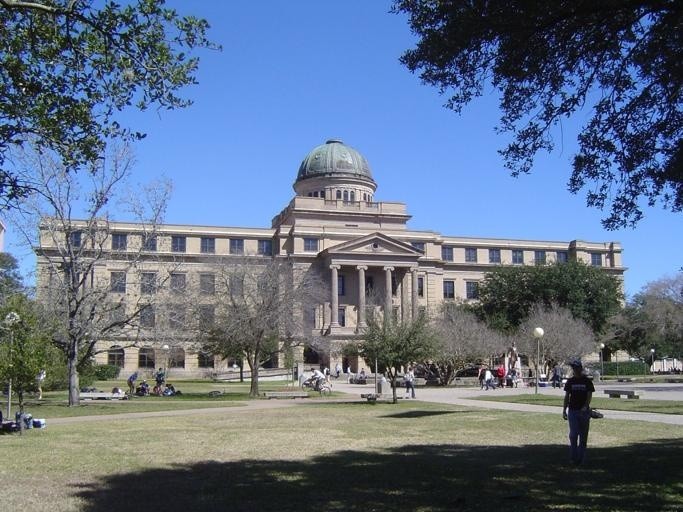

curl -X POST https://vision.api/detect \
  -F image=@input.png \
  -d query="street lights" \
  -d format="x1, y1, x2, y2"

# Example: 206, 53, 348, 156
534, 327, 544, 394
651, 349, 654, 374
600, 343, 605, 382
5, 311, 21, 420
162, 344, 170, 383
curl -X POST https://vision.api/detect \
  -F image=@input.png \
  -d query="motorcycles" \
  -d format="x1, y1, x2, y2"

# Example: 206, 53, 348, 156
302, 377, 332, 395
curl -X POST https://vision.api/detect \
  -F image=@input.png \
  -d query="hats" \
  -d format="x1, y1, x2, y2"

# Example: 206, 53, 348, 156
569, 359, 582, 369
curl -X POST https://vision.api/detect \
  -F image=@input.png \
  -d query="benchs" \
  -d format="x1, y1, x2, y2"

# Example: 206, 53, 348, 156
360, 393, 410, 402
612, 377, 632, 382
603, 388, 645, 400
260, 391, 308, 399
78, 390, 124, 402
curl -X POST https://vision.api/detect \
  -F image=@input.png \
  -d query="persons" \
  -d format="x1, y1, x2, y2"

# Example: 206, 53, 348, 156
506, 340, 518, 367
562, 360, 603, 463
402, 365, 416, 399
551, 363, 563, 389
346, 363, 352, 384
323, 365, 332, 387
358, 367, 368, 379
127, 366, 183, 397
478, 363, 516, 392
335, 362, 340, 378
36, 368, 45, 401
310, 367, 325, 390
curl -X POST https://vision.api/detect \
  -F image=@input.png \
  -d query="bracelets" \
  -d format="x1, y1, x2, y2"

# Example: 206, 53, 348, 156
563, 407, 566, 410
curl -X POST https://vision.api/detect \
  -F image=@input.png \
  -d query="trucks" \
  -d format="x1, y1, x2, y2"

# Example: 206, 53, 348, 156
650, 358, 674, 374
